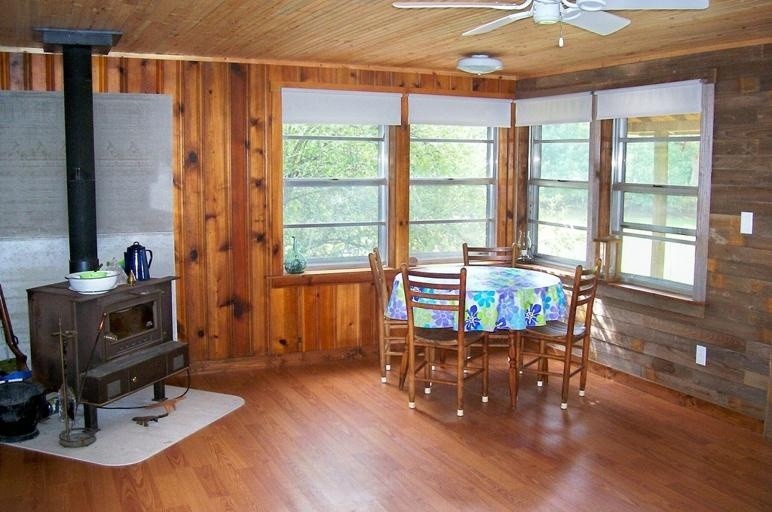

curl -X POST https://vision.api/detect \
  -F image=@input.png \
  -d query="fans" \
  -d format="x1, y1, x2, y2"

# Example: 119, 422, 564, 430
390, 0, 711, 40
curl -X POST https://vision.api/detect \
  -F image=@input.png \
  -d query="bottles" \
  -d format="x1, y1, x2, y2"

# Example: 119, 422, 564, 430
515, 229, 535, 262
284, 235, 307, 275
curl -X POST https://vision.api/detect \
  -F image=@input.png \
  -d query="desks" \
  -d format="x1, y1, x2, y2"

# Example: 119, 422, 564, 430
385, 264, 567, 410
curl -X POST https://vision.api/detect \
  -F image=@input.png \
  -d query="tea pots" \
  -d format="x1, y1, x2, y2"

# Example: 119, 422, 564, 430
124, 241, 153, 281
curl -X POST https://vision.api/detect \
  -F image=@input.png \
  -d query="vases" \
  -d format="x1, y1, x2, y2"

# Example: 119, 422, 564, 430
284, 237, 305, 275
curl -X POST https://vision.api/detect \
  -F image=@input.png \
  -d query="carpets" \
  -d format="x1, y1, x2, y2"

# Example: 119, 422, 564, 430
1, 382, 245, 468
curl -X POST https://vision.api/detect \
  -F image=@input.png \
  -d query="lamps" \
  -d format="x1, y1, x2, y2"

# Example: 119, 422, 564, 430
512, 228, 538, 261
452, 54, 506, 77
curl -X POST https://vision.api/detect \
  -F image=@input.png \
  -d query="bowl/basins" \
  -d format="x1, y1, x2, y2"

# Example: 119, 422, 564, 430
79, 272, 107, 278
64, 270, 120, 295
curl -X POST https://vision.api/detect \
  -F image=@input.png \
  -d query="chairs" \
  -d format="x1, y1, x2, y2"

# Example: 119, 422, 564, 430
516, 256, 605, 412
366, 245, 439, 395
400, 263, 491, 416
453, 242, 521, 377
